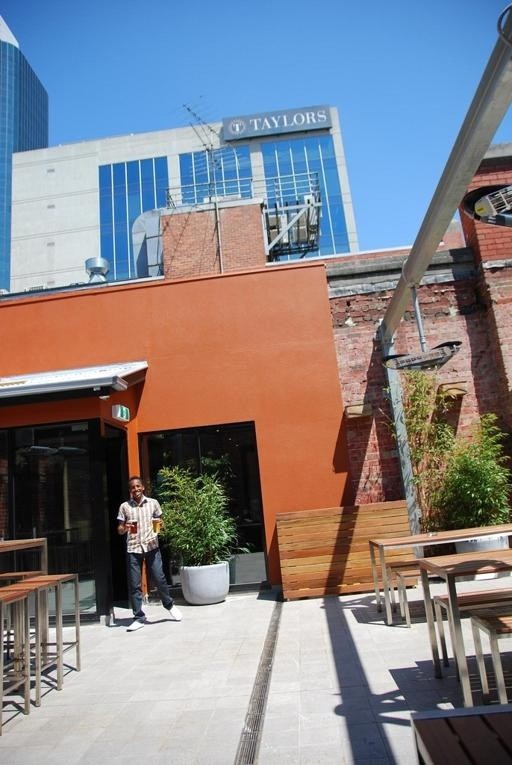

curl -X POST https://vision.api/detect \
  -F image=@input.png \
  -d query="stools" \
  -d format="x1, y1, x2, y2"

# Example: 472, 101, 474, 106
1, 570, 81, 737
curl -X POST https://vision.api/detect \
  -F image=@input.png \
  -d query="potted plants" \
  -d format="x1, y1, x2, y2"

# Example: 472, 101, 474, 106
154, 462, 250, 606
413, 411, 511, 581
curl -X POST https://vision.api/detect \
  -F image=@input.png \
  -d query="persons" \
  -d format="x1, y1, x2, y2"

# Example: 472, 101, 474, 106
116, 476, 183, 632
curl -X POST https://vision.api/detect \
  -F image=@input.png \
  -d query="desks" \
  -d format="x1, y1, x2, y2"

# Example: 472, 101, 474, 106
0, 537, 49, 667
418, 550, 512, 708
367, 523, 512, 626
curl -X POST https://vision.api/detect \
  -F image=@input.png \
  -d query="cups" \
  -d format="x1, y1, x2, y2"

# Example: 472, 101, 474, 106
126, 520, 137, 534
151, 517, 161, 534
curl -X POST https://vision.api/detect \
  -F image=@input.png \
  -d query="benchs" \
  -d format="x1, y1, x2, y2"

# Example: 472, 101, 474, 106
410, 582, 511, 763
386, 557, 512, 627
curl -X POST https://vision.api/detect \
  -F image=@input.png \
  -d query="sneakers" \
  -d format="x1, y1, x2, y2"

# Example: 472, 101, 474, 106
169, 604, 183, 621
126, 620, 145, 631
